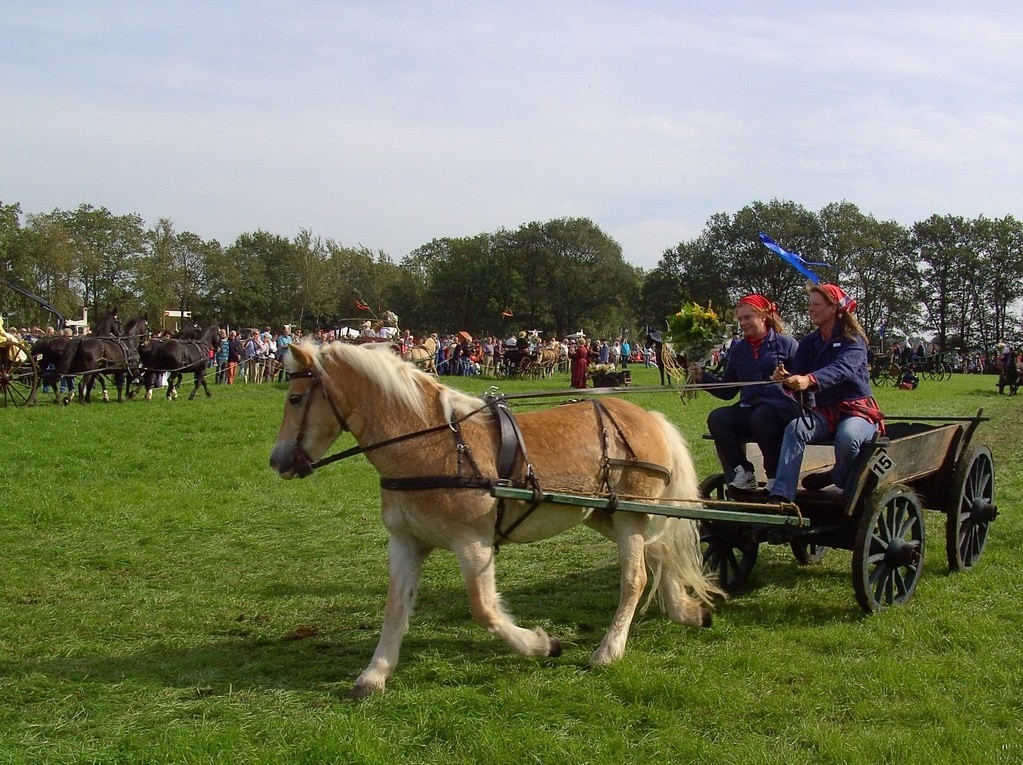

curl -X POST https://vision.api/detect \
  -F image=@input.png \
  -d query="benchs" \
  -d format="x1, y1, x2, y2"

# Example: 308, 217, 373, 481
883, 415, 994, 422
702, 432, 893, 451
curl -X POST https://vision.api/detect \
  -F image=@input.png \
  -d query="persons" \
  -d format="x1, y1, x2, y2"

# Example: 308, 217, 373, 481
769, 284, 885, 503
0, 316, 171, 394
687, 295, 815, 491
360, 324, 629, 389
209, 328, 335, 387
888, 341, 925, 359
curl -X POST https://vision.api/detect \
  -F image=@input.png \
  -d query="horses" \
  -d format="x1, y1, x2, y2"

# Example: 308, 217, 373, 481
269, 333, 729, 703
35, 304, 222, 407
1005, 352, 1021, 396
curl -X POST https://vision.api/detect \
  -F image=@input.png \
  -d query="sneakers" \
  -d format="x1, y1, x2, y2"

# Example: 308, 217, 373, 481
763, 477, 775, 494
728, 464, 758, 491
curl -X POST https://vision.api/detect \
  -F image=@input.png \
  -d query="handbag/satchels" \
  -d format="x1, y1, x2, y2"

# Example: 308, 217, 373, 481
255, 354, 262, 362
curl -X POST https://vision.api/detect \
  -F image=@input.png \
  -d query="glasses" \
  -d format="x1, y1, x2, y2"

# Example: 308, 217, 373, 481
297, 330, 302, 333
253, 333, 258, 335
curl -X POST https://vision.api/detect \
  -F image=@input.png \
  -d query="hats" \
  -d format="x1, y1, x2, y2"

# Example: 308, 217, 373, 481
519, 331, 527, 339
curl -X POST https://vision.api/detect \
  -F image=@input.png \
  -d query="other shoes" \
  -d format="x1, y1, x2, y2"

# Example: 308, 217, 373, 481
801, 473, 833, 490
766, 495, 790, 505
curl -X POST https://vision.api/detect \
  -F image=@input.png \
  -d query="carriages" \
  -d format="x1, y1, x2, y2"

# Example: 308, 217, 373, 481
493, 343, 561, 379
270, 334, 997, 701
996, 351, 1023, 397
866, 349, 952, 389
335, 318, 440, 378
0, 309, 219, 411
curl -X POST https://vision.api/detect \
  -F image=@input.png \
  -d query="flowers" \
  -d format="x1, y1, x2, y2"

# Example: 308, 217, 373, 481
586, 363, 617, 377
647, 299, 730, 402
